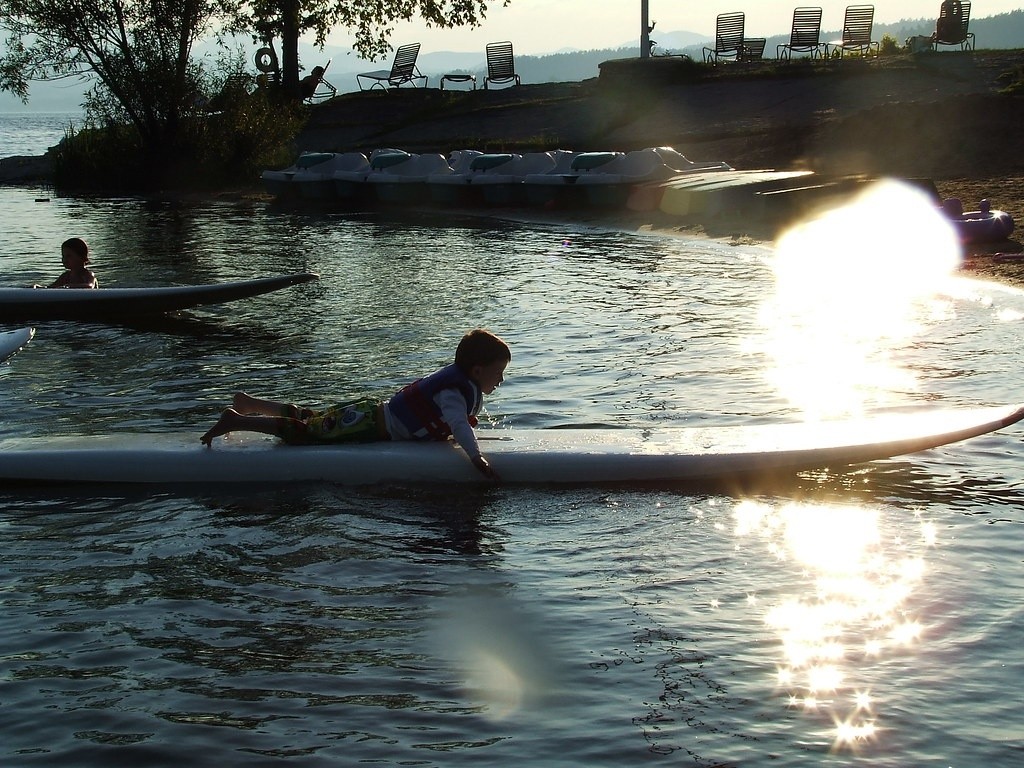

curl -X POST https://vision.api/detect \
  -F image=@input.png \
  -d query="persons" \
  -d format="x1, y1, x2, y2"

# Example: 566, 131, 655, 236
47, 238, 98, 289
200, 330, 511, 478
299, 66, 323, 94
937, 0, 962, 41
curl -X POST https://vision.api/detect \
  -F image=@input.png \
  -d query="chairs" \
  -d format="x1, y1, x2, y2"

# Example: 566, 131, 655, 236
831, 5, 879, 59
440, 75, 476, 92
356, 43, 428, 95
735, 38, 767, 63
252, 73, 279, 97
187, 72, 251, 116
301, 60, 337, 105
484, 41, 521, 91
703, 12, 752, 65
776, 7, 828, 61
929, 0, 976, 50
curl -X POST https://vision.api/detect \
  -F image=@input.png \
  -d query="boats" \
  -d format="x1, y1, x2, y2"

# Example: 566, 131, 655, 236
262, 148, 732, 185
